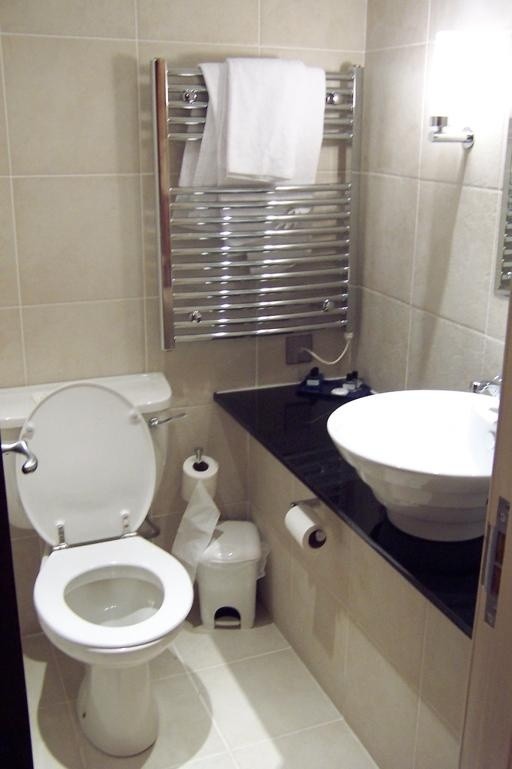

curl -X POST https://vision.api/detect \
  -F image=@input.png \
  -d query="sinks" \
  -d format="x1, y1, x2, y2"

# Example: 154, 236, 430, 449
327, 389, 499, 542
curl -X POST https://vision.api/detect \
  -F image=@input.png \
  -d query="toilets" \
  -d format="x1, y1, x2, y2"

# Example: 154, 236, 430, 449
2, 369, 196, 758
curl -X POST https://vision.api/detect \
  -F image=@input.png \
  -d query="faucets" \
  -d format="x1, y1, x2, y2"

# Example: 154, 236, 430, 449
486, 404, 499, 441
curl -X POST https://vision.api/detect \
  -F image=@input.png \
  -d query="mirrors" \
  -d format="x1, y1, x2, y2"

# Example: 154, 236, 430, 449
491, 114, 512, 299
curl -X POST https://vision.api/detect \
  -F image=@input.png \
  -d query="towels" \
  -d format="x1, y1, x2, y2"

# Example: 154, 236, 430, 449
179, 60, 326, 271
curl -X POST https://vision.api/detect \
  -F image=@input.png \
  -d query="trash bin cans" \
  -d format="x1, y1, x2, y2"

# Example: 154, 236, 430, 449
196, 520, 262, 631
